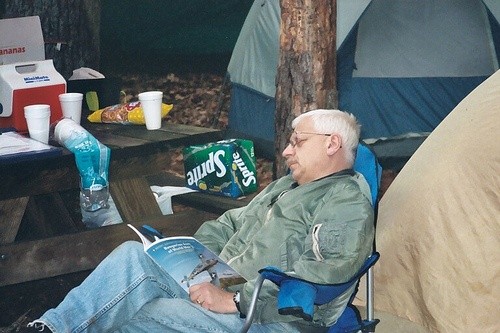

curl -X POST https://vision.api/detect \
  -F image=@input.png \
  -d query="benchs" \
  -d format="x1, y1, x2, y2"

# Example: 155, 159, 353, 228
0, 210, 223, 288
145, 172, 258, 214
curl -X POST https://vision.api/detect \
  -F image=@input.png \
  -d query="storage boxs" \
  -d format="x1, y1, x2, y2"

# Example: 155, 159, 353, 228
183, 138, 259, 199
0, 59, 67, 134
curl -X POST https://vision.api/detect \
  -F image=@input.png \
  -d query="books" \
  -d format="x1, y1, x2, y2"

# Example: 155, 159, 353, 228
127, 223, 247, 295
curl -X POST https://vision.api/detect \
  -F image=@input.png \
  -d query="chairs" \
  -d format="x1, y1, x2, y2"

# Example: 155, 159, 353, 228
236, 140, 382, 333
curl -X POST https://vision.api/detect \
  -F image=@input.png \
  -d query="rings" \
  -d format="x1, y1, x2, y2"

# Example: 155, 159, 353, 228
196, 298, 201, 304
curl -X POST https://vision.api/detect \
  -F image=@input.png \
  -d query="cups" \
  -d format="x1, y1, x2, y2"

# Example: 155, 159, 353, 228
58, 93, 83, 126
24, 104, 51, 145
138, 90, 163, 131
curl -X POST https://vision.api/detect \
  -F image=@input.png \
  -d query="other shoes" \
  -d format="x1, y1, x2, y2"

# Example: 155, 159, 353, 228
18, 321, 53, 333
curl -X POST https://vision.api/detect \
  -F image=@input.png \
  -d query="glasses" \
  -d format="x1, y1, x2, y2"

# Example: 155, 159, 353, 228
284, 131, 342, 149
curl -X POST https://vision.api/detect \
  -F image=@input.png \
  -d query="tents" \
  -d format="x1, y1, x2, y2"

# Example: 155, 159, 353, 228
222, 0, 500, 161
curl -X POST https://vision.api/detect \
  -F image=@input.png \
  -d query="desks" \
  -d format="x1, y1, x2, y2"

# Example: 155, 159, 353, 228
0, 123, 223, 327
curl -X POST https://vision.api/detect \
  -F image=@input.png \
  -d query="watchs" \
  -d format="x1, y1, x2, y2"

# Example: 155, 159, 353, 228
233, 290, 241, 312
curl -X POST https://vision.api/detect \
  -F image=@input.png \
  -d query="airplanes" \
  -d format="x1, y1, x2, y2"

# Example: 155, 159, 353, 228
180, 246, 223, 289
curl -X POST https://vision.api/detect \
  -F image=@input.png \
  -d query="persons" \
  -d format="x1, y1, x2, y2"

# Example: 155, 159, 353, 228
18, 109, 375, 333
